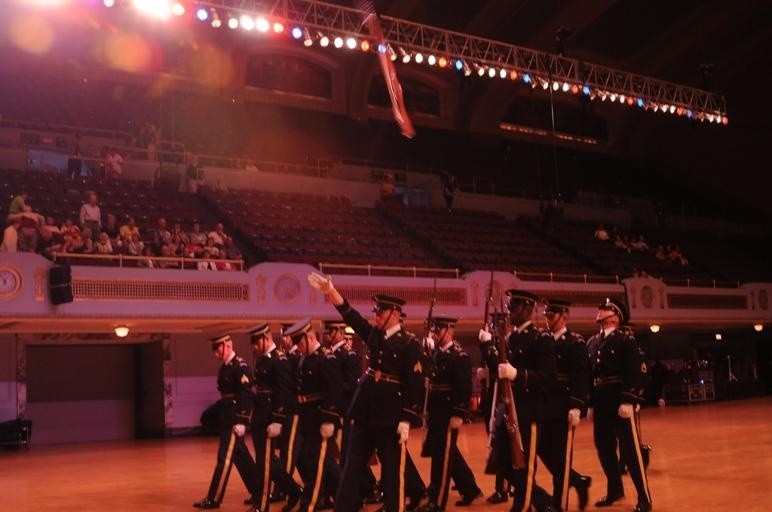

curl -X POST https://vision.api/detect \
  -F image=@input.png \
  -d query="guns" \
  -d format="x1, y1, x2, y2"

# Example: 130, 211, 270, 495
414, 277, 436, 416
479, 269, 494, 413
495, 288, 526, 469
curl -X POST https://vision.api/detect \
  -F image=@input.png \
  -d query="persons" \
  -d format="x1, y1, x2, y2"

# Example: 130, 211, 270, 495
422, 316, 481, 511
478, 291, 592, 512
440, 170, 458, 216
1, 110, 246, 271
658, 360, 677, 404
192, 330, 270, 511
586, 298, 652, 509
309, 271, 429, 512
593, 221, 689, 267
381, 172, 405, 204
242, 320, 379, 511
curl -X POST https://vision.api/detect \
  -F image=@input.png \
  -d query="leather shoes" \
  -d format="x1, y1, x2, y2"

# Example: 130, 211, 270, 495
641, 444, 650, 469
595, 493, 625, 508
244, 462, 439, 512
193, 498, 218, 508
451, 476, 592, 512
632, 501, 652, 512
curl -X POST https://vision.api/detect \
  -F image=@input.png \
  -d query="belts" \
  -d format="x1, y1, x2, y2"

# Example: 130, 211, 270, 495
255, 385, 272, 393
429, 383, 457, 393
297, 393, 320, 404
220, 392, 240, 400
555, 372, 570, 382
592, 375, 626, 387
366, 367, 402, 385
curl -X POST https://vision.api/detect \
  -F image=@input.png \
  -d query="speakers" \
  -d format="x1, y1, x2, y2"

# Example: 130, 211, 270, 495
51, 286, 73, 305
50, 266, 71, 284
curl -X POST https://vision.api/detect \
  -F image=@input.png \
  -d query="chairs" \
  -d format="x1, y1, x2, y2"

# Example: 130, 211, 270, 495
0, 159, 771, 288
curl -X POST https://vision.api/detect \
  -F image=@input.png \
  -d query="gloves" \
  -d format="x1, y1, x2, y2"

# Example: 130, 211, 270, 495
450, 416, 463, 430
397, 421, 410, 444
479, 329, 492, 343
618, 403, 634, 419
307, 272, 333, 294
266, 423, 282, 438
319, 422, 335, 438
568, 407, 581, 426
232, 424, 246, 437
498, 359, 518, 381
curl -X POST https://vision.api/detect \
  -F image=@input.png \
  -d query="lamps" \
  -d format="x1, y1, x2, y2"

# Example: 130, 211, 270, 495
209, 1, 727, 130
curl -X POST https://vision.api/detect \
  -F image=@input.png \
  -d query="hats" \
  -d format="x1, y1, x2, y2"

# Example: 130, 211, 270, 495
279, 320, 355, 344
600, 296, 629, 324
371, 294, 406, 312
209, 331, 232, 351
398, 312, 407, 322
489, 289, 571, 322
430, 317, 458, 331
244, 321, 270, 344
